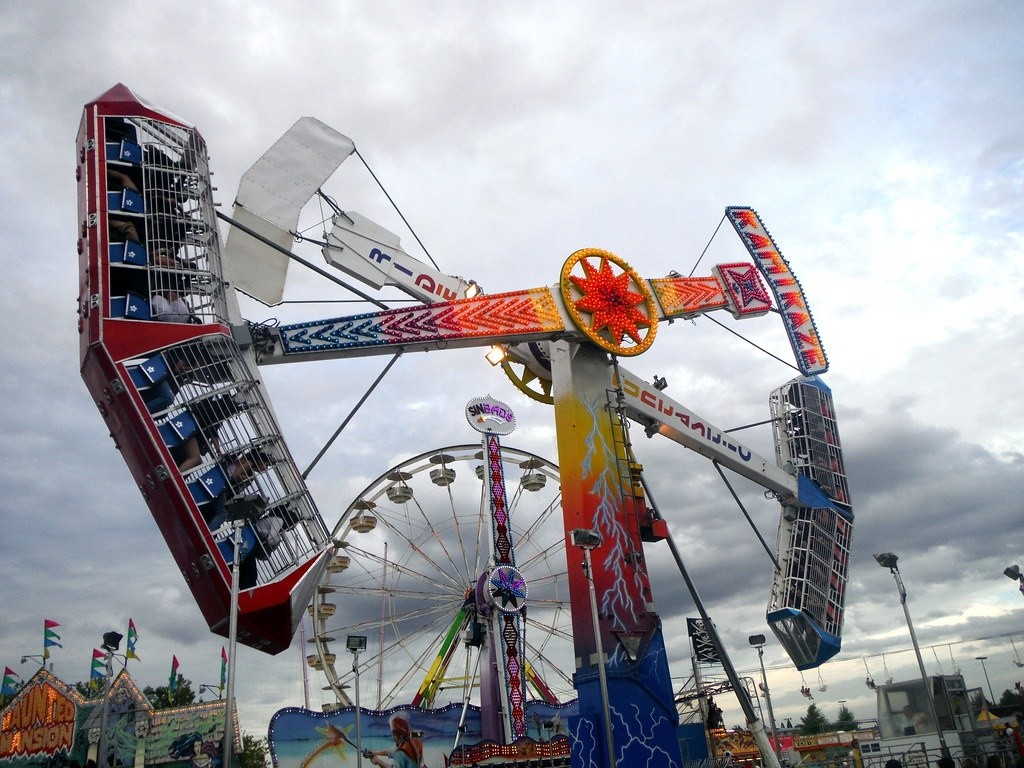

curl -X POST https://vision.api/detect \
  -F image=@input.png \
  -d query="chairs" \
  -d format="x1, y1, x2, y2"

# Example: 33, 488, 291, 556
106, 117, 287, 588
786, 383, 852, 636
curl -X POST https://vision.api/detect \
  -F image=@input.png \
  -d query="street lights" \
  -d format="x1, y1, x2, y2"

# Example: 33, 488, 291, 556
98, 631, 123, 767
839, 700, 846, 713
975, 657, 1001, 721
1005, 564, 1023, 594
747, 633, 784, 768
345, 635, 367, 768
873, 553, 953, 766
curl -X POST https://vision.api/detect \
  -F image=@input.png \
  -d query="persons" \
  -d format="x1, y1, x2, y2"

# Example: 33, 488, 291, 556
934, 722, 1024, 768
866, 678, 876, 689
102, 118, 206, 330
782, 506, 851, 650
786, 385, 849, 506
848, 737, 864, 768
722, 749, 734, 768
801, 687, 814, 700
156, 341, 298, 589
900, 704, 931, 735
